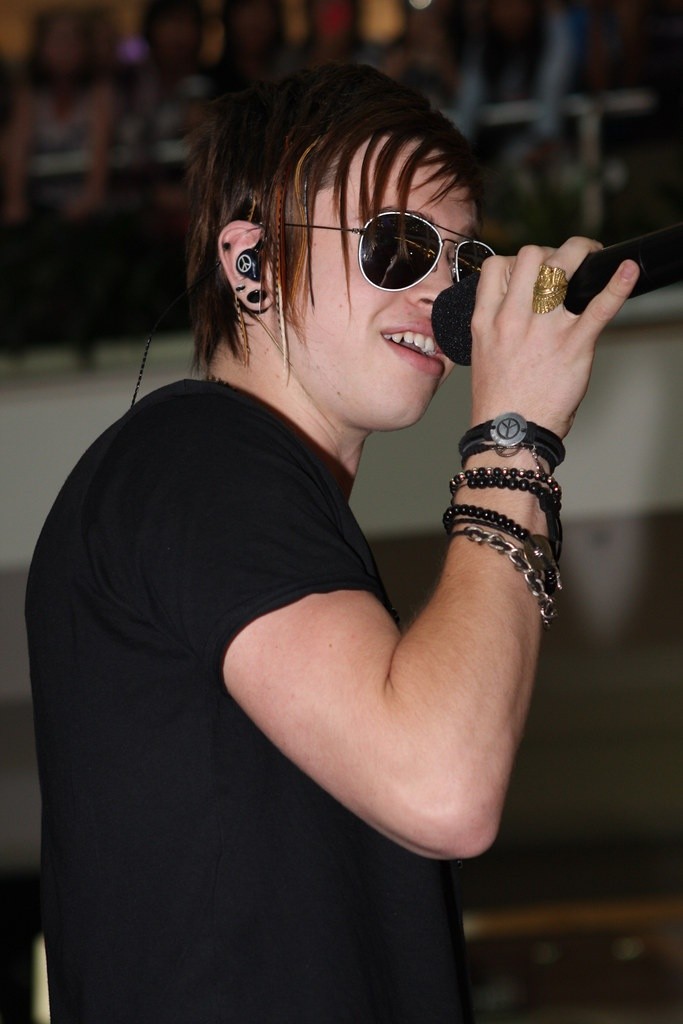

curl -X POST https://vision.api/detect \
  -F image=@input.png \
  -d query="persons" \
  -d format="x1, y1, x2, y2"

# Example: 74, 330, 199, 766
0, 0, 682, 370
23, 57, 641, 1024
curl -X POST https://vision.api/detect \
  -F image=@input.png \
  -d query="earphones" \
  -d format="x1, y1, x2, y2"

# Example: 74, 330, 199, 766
236, 241, 263, 281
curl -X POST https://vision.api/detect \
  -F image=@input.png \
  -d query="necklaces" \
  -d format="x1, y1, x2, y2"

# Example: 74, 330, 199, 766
206, 376, 229, 384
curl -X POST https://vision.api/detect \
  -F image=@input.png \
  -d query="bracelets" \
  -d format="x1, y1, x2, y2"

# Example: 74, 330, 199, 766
441, 412, 566, 624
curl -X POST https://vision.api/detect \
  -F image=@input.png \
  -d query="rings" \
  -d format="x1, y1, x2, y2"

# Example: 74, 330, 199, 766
530, 265, 569, 315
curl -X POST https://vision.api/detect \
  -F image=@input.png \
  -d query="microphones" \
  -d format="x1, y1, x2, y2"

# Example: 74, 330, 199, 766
431, 224, 683, 366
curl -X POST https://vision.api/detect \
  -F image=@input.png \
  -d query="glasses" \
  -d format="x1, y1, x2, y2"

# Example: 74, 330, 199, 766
273, 212, 497, 293
